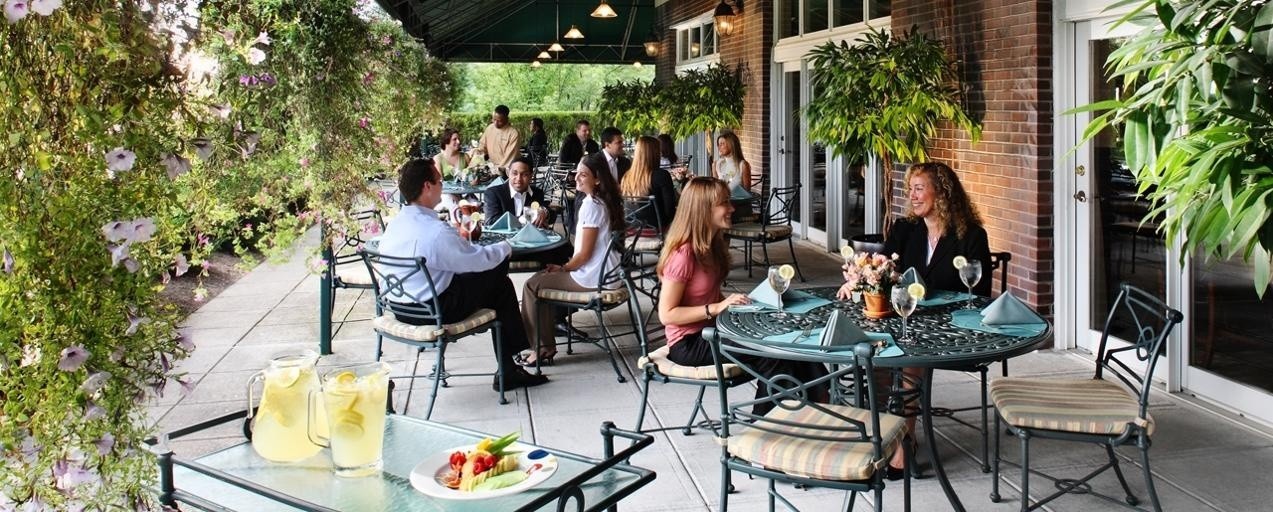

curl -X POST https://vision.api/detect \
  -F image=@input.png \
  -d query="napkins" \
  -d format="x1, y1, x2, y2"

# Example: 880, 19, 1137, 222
980, 290, 1043, 325
730, 185, 752, 199
508, 222, 550, 242
817, 310, 872, 353
489, 211, 523, 232
901, 265, 928, 291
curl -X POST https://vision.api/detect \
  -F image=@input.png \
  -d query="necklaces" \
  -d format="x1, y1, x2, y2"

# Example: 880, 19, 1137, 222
931, 234, 940, 243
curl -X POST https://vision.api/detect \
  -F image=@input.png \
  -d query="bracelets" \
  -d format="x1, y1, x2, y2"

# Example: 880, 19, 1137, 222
703, 303, 713, 321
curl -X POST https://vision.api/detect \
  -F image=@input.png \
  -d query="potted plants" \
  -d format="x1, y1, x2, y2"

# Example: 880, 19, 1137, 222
787, 18, 984, 259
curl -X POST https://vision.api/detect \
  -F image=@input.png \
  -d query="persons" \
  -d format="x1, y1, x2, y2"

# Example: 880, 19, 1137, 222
431, 127, 480, 227
556, 120, 597, 221
522, 118, 549, 168
481, 157, 559, 230
471, 104, 517, 180
511, 153, 625, 368
870, 161, 992, 482
709, 132, 752, 250
654, 176, 831, 424
372, 157, 550, 391
617, 134, 675, 230
657, 134, 677, 168
570, 126, 631, 227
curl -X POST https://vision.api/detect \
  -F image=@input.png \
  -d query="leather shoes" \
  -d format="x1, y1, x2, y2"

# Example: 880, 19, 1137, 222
555, 321, 589, 341
886, 440, 918, 481
493, 366, 549, 391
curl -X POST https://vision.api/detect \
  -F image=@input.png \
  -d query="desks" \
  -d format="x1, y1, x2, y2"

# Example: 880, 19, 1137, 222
137, 379, 656, 511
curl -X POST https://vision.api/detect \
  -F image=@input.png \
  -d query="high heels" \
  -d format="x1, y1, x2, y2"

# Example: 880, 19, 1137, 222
513, 349, 558, 367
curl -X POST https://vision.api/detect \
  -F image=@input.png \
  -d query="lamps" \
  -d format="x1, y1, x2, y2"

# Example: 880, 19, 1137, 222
528, 0, 744, 71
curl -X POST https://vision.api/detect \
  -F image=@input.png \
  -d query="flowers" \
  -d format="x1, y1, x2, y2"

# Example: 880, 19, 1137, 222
670, 166, 687, 181
834, 252, 903, 301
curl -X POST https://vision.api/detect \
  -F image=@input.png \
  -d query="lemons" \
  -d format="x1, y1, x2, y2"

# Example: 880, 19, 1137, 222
841, 245, 854, 259
458, 199, 468, 207
530, 201, 539, 210
335, 372, 366, 433
908, 283, 926, 299
952, 255, 967, 270
255, 367, 311, 425
778, 264, 795, 280
471, 212, 481, 222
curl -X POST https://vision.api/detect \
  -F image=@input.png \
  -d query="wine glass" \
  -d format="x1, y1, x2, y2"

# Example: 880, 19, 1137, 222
767, 266, 791, 320
891, 284, 916, 342
958, 260, 982, 311
461, 217, 476, 248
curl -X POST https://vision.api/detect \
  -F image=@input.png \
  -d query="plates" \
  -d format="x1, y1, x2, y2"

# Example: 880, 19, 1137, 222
409, 444, 558, 501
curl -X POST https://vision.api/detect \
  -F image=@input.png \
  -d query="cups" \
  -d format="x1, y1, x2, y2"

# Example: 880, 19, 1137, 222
306, 362, 391, 479
453, 205, 481, 240
244, 351, 329, 465
524, 207, 538, 223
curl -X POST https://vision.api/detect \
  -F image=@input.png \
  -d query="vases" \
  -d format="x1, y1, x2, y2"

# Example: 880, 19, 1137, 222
862, 291, 893, 320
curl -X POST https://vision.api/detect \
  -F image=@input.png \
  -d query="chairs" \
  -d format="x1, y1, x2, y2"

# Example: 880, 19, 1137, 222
700, 327, 915, 511
733, 173, 766, 222
507, 261, 545, 274
679, 154, 692, 168
931, 252, 1014, 471
722, 183, 806, 288
618, 194, 664, 290
320, 210, 386, 323
372, 178, 409, 210
357, 247, 507, 422
519, 141, 579, 238
617, 262, 758, 465
987, 284, 1184, 512
533, 222, 648, 383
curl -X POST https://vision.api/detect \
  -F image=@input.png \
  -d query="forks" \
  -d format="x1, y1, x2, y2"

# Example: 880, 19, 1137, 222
789, 324, 818, 344
